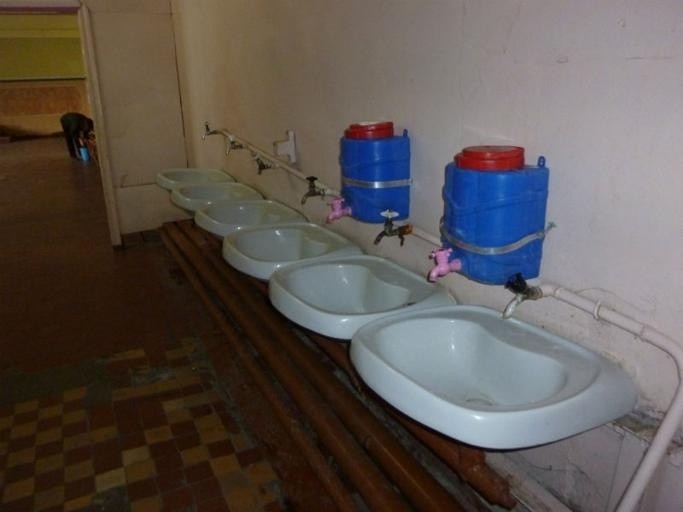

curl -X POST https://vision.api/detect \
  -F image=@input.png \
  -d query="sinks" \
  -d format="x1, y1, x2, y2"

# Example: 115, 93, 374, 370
194, 200, 308, 242
221, 223, 365, 283
266, 254, 457, 343
348, 302, 638, 454
155, 167, 235, 194
170, 183, 265, 213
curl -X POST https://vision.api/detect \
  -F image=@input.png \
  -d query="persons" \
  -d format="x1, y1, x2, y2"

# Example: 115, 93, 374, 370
60, 112, 93, 160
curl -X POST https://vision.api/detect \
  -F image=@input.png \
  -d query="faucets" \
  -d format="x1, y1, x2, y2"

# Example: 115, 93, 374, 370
225, 134, 248, 157
201, 121, 218, 142
325, 198, 352, 224
503, 272, 542, 318
251, 152, 273, 175
301, 176, 325, 205
428, 248, 461, 282
374, 210, 411, 246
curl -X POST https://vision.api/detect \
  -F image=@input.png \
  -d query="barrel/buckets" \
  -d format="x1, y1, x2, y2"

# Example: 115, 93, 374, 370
80, 147, 90, 161
338, 120, 411, 225
441, 144, 551, 286
